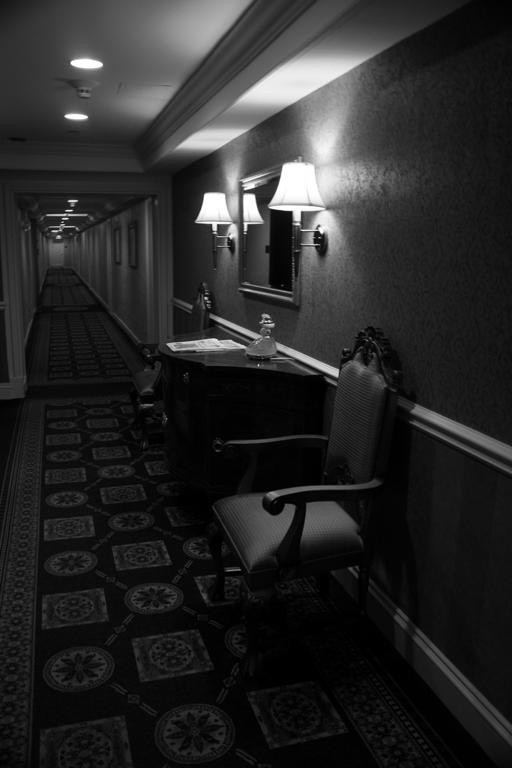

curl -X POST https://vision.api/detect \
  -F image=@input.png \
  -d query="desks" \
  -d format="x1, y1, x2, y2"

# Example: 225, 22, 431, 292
158, 331, 325, 507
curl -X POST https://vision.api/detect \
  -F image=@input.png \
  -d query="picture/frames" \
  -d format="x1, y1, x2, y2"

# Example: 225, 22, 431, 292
127, 219, 137, 269
113, 225, 121, 264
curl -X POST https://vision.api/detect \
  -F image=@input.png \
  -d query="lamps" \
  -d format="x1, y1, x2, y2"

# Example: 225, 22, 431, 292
267, 156, 328, 256
195, 190, 236, 266
243, 192, 264, 252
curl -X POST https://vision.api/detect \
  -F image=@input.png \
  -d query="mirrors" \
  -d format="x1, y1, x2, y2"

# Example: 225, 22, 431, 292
238, 156, 305, 307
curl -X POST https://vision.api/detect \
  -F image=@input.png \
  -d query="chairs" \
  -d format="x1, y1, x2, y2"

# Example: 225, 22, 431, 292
209, 324, 400, 679
132, 281, 214, 450
